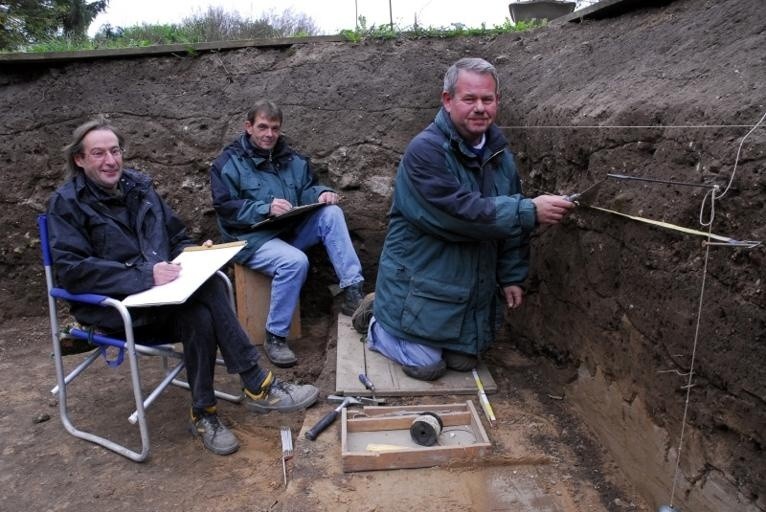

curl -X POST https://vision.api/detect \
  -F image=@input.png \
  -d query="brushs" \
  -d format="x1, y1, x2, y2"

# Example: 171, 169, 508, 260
280, 423, 296, 486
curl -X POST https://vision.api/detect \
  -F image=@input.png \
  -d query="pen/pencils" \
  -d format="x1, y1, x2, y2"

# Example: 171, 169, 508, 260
269, 194, 276, 201
152, 251, 169, 262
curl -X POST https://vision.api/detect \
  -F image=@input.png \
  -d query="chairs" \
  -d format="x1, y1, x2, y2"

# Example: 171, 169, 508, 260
37, 212, 247, 463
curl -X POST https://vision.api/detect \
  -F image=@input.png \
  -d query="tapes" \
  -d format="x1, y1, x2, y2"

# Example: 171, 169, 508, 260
409, 412, 443, 446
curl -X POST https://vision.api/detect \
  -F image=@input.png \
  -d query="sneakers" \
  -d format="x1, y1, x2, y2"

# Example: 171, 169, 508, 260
342, 282, 365, 316
190, 405, 239, 454
240, 368, 320, 413
263, 329, 297, 368
352, 292, 375, 334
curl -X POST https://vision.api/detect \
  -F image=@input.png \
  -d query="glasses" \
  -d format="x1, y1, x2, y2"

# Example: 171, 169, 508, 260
83, 148, 126, 158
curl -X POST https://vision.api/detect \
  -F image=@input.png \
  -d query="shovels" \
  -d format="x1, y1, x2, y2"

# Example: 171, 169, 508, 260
534, 182, 602, 238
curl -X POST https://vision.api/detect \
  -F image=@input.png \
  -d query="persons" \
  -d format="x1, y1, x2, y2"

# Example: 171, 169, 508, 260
44, 116, 323, 453
208, 100, 375, 365
350, 57, 580, 380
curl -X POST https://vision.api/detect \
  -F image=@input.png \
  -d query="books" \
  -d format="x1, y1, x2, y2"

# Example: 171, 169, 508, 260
119, 240, 247, 308
249, 196, 346, 232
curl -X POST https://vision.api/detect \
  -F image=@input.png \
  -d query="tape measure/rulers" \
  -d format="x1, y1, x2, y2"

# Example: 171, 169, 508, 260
586, 203, 762, 248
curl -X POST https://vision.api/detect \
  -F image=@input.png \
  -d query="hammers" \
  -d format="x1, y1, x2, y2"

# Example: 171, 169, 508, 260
305, 395, 363, 442
357, 375, 387, 407
